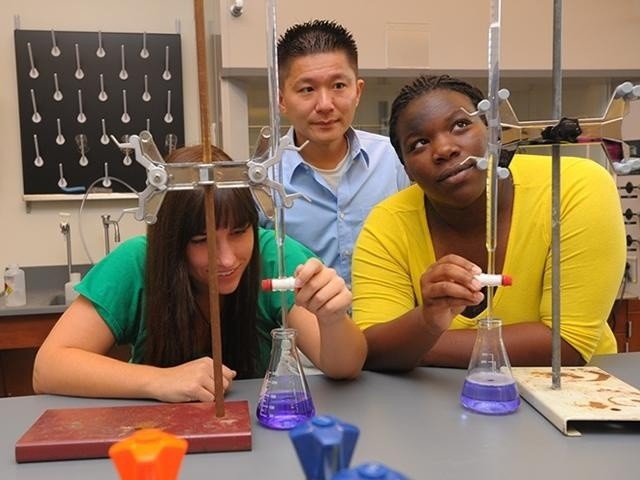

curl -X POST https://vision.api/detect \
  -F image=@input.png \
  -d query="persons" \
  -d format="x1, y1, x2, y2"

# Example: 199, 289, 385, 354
32, 144, 368, 403
252, 19, 412, 370
351, 74, 627, 372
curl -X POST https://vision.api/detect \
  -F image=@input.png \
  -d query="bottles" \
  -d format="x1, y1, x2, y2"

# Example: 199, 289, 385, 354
256, 329, 316, 430
64, 272, 82, 308
4, 264, 27, 307
458, 319, 520, 415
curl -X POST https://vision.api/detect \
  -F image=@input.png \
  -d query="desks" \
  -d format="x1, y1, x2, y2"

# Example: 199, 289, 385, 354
0, 353, 640, 480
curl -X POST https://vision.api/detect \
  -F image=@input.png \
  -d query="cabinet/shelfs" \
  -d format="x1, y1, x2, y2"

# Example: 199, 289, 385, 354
607, 298, 640, 353
